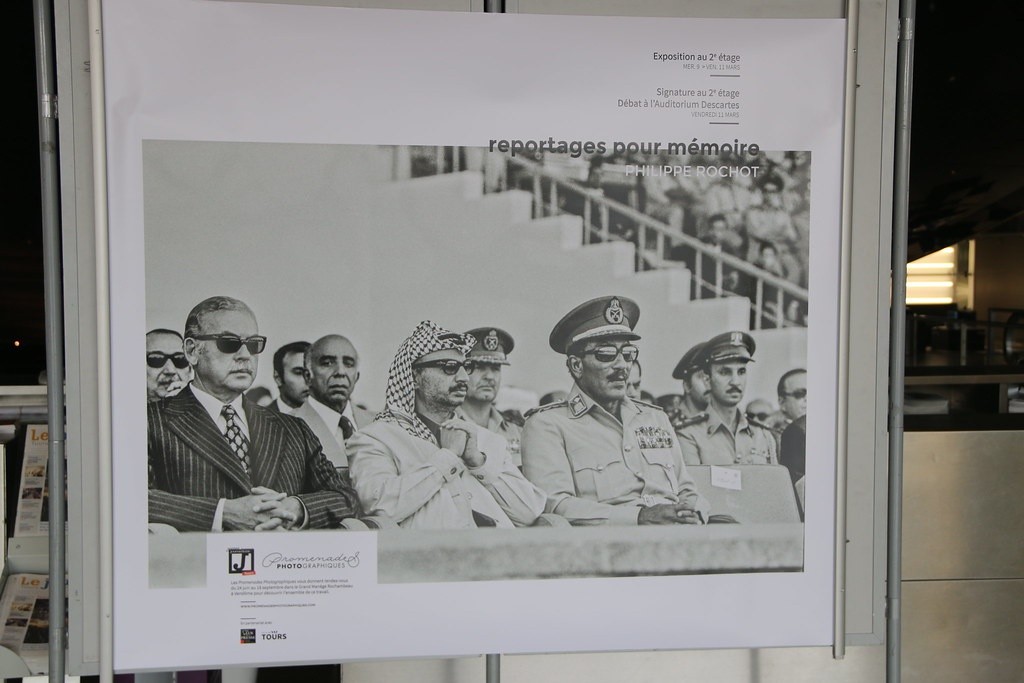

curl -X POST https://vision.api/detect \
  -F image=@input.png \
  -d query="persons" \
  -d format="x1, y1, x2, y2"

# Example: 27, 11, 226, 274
147, 296, 807, 532
559, 149, 810, 326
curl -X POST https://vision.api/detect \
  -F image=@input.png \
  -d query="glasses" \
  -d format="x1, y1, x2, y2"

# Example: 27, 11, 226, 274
572, 344, 639, 363
190, 333, 267, 354
785, 388, 807, 398
147, 351, 189, 368
411, 359, 474, 375
747, 412, 768, 421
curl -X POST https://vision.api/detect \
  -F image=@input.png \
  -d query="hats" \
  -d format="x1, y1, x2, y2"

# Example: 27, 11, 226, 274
461, 327, 514, 365
691, 331, 756, 370
549, 296, 641, 355
671, 342, 708, 378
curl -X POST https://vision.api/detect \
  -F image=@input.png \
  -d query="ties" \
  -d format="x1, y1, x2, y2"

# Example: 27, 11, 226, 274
221, 404, 251, 477
339, 416, 354, 446
